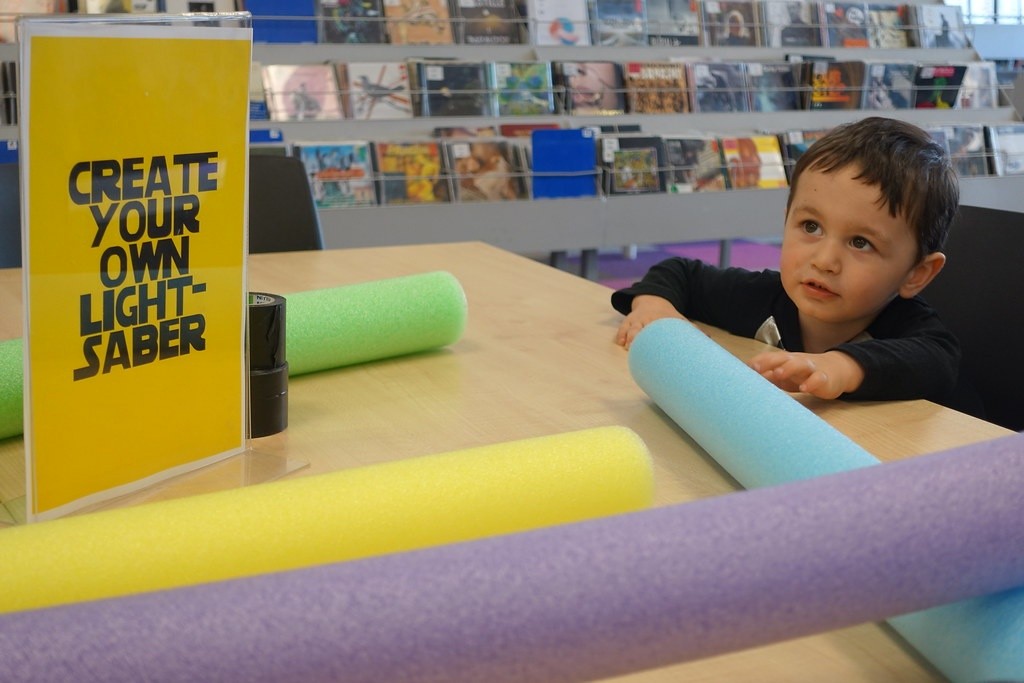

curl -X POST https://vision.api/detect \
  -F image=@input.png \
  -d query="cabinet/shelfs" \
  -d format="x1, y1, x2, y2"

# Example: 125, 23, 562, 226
0, 10, 1024, 284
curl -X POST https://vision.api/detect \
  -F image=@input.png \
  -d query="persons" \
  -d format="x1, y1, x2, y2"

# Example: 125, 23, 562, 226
611, 117, 962, 401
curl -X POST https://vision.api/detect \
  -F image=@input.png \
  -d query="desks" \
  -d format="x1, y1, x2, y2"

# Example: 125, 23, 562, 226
0, 238, 1024, 683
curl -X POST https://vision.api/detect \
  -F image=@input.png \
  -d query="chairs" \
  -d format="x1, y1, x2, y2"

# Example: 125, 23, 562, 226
249, 155, 325, 253
923, 205, 1024, 434
